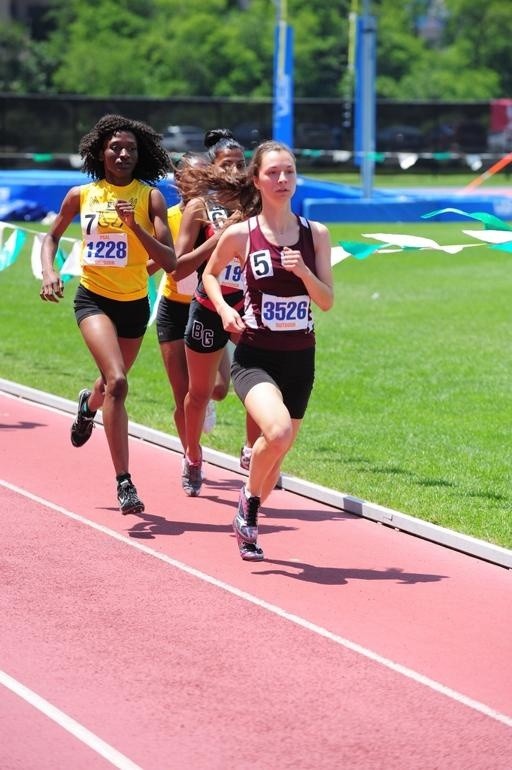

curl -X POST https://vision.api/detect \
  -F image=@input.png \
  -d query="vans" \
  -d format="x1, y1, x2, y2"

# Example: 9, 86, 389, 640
428, 122, 488, 153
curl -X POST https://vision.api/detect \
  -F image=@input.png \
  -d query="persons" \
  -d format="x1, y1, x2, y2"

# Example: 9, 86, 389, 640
169, 126, 248, 497
204, 140, 335, 565
37, 114, 178, 516
147, 150, 231, 461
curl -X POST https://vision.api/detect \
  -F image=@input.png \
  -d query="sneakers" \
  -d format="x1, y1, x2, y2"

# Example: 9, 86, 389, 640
233, 485, 264, 560
204, 401, 216, 433
241, 447, 250, 470
182, 445, 205, 497
71, 389, 97, 447
118, 478, 144, 515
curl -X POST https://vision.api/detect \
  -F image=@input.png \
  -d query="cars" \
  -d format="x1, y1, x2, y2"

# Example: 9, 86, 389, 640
297, 122, 333, 149
234, 122, 269, 149
160, 124, 207, 152
377, 126, 424, 150
